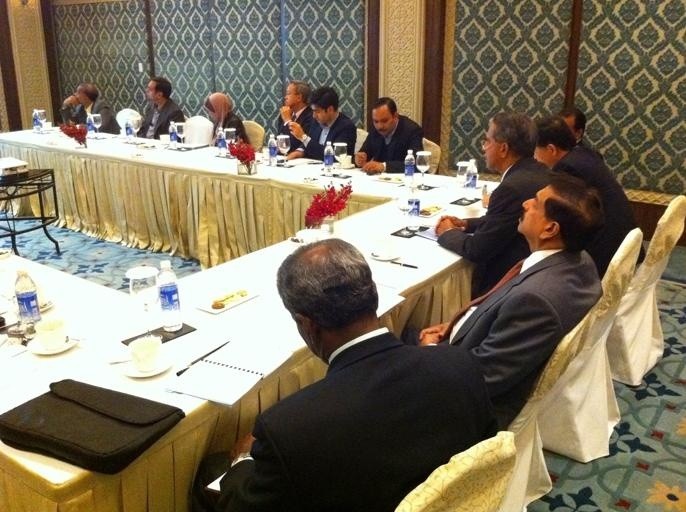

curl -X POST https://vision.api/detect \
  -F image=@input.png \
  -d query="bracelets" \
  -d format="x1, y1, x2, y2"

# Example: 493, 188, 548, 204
234, 451, 251, 458
300, 135, 308, 144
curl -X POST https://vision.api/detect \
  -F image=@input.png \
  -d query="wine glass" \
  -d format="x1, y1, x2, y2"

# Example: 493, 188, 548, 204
416, 151, 432, 191
87, 112, 95, 137
333, 142, 347, 177
456, 162, 469, 190
174, 122, 187, 150
130, 120, 141, 143
397, 191, 416, 237
37, 110, 47, 132
91, 114, 102, 136
224, 127, 237, 159
276, 135, 290, 168
127, 266, 161, 343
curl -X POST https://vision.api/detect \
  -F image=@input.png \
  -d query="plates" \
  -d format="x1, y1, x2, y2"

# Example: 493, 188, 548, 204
120, 343, 173, 382
25, 333, 81, 356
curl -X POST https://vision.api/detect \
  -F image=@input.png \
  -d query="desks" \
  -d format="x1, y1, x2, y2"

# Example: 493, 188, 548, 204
0, 125, 504, 267
0, 169, 62, 255
0, 197, 502, 511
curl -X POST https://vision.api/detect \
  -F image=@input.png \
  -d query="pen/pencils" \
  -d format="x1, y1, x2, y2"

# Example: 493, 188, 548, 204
391, 261, 418, 269
191, 342, 229, 365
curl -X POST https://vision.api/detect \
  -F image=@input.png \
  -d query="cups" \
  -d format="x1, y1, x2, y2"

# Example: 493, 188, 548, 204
127, 334, 163, 371
33, 317, 67, 347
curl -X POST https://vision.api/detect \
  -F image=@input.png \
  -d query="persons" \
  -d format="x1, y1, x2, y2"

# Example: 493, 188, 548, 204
59, 83, 118, 135
531, 114, 647, 280
283, 87, 356, 163
276, 79, 311, 150
135, 76, 185, 141
350, 97, 425, 176
434, 111, 553, 301
559, 107, 587, 143
417, 172, 603, 407
189, 237, 500, 511
201, 91, 251, 150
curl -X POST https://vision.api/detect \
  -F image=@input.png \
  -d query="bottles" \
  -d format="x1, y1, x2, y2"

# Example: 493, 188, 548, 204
125, 119, 131, 142
268, 134, 277, 166
168, 120, 177, 148
12, 267, 42, 330
217, 128, 227, 157
405, 149, 416, 188
324, 142, 333, 177
157, 258, 183, 335
406, 192, 422, 233
34, 110, 42, 132
466, 158, 477, 190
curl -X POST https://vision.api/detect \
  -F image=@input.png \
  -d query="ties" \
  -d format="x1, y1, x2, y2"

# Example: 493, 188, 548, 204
439, 259, 525, 344
290, 112, 296, 121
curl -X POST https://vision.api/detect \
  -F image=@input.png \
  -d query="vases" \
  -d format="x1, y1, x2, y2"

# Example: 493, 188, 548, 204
236, 162, 257, 175
320, 216, 337, 240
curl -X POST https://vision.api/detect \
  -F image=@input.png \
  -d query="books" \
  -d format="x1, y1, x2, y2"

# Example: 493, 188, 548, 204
166, 339, 295, 410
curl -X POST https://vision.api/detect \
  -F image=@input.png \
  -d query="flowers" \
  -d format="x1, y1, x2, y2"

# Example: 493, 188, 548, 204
59, 122, 88, 148
227, 138, 255, 174
304, 182, 354, 228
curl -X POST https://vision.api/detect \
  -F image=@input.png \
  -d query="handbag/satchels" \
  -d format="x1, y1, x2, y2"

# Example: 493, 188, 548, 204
0, 379, 185, 474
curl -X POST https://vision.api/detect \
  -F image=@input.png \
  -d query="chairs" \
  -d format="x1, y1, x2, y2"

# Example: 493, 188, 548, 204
595, 229, 645, 322
503, 271, 615, 509
604, 195, 686, 386
421, 138, 442, 174
242, 120, 266, 153
395, 428, 518, 511
